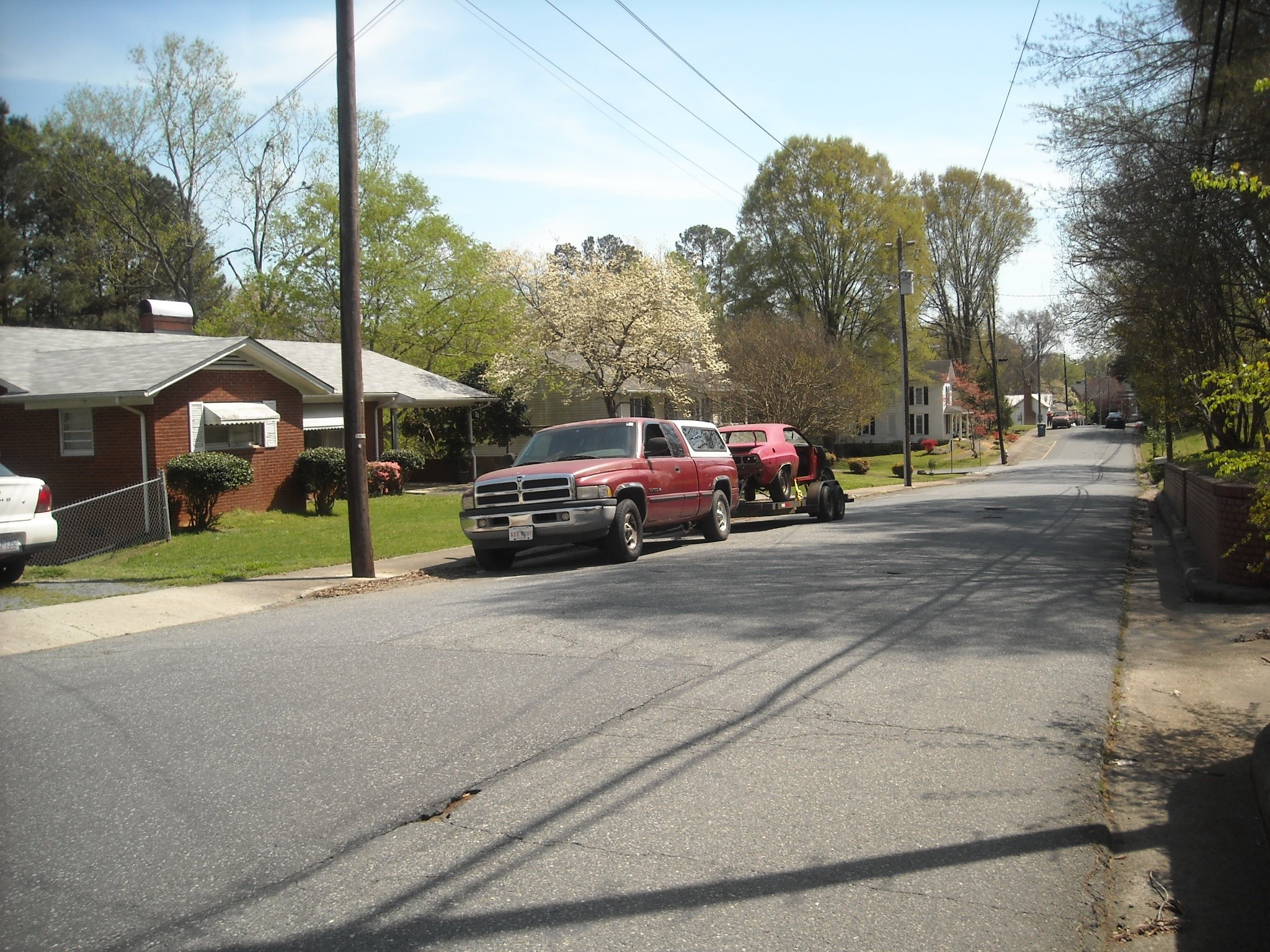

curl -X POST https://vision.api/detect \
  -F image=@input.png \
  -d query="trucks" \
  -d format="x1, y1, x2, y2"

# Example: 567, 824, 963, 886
1067, 410, 1078, 424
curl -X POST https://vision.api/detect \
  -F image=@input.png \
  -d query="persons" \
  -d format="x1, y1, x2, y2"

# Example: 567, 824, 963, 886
1047, 411, 1052, 426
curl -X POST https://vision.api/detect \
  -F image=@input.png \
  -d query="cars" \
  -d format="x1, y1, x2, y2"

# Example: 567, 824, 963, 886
1106, 412, 1125, 429
715, 423, 836, 502
0, 462, 57, 582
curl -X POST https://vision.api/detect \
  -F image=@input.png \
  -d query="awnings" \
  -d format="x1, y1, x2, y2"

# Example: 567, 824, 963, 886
203, 402, 280, 425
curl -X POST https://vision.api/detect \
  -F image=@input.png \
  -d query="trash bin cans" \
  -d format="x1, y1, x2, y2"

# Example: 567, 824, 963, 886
1037, 424, 1046, 437
457, 459, 472, 483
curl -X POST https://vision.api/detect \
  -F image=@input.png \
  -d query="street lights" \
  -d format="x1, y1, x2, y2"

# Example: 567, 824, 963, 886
993, 333, 1016, 375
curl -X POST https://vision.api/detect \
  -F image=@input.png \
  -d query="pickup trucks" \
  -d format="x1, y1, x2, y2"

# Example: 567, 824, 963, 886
1051, 411, 1072, 429
459, 417, 740, 572
